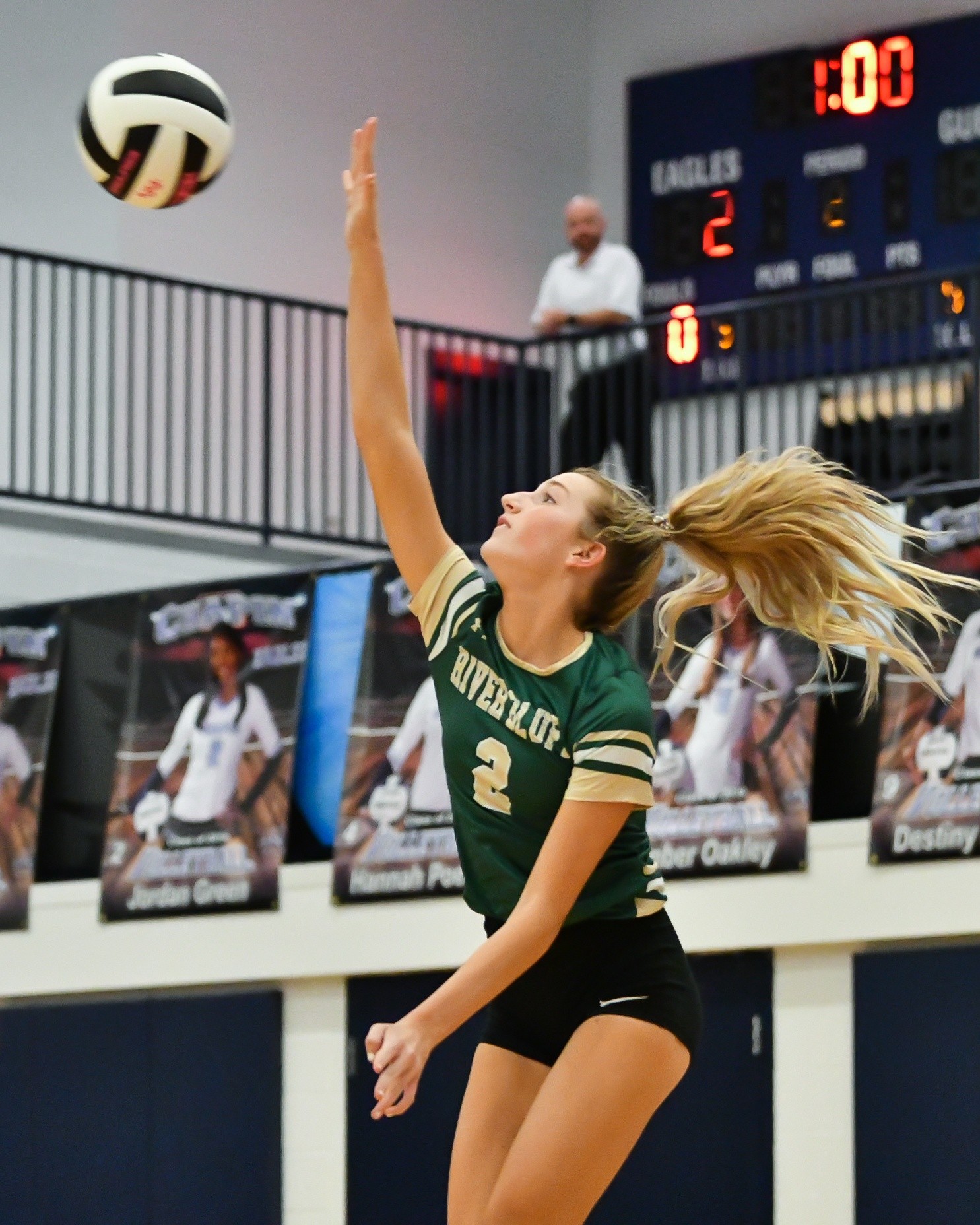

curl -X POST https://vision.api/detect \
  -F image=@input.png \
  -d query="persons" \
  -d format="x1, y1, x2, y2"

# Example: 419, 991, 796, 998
529, 193, 660, 509
341, 675, 455, 829
0, 723, 30, 891
106, 620, 284, 848
878, 612, 978, 775
655, 586, 800, 807
340, 114, 979, 1225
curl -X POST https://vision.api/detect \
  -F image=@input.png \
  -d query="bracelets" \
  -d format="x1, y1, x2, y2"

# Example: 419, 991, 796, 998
566, 313, 578, 329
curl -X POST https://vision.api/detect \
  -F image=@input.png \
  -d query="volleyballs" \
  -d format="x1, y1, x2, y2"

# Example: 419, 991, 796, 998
73, 51, 239, 209
